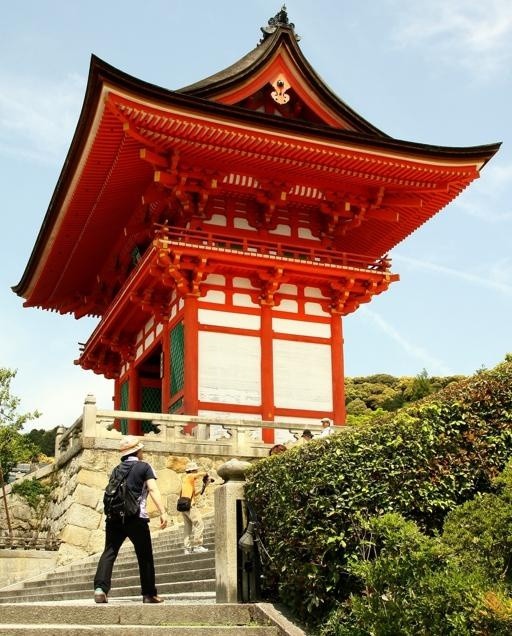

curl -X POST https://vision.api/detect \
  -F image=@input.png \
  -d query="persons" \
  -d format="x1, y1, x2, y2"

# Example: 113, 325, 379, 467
180, 461, 214, 555
93, 435, 168, 605
292, 429, 315, 448
318, 417, 335, 439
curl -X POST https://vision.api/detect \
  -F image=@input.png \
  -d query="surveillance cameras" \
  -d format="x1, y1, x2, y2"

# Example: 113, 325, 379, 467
277, 80, 284, 86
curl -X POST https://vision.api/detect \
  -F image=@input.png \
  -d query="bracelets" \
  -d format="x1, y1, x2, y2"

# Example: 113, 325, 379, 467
159, 510, 167, 515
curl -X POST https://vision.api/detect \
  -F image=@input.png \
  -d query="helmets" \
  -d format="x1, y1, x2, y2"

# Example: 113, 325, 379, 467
184, 462, 200, 472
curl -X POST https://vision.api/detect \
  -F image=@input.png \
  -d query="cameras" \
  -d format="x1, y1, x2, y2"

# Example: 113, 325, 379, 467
203, 475, 214, 483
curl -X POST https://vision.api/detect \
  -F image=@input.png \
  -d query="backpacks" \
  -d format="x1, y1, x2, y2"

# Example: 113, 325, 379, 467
102, 464, 147, 522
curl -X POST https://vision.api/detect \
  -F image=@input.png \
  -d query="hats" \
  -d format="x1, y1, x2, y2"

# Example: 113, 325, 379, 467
117, 434, 146, 461
301, 429, 315, 438
320, 417, 330, 422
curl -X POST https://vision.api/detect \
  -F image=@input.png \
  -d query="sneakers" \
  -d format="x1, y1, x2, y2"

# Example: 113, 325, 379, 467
94, 587, 108, 603
184, 545, 209, 555
142, 594, 165, 603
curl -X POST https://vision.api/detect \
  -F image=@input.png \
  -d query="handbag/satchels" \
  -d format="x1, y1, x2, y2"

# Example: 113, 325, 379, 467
177, 496, 191, 512
